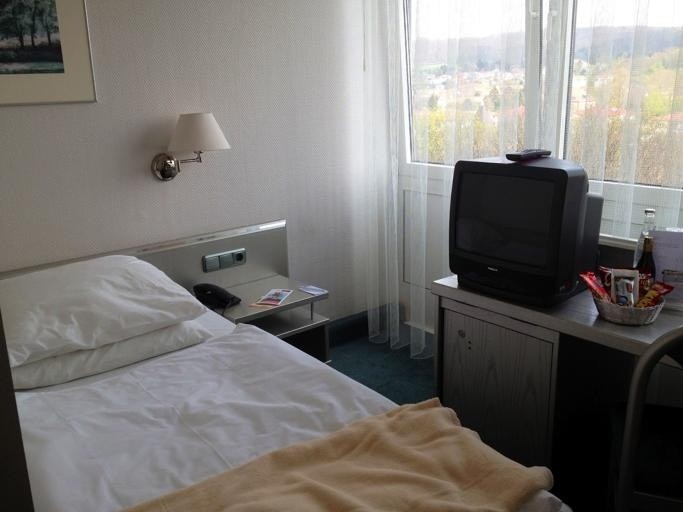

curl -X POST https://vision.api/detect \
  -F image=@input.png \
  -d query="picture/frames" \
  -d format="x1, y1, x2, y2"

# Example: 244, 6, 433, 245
0, 0, 99, 105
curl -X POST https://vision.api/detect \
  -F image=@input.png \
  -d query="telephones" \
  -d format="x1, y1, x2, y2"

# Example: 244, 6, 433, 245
193, 283, 242, 310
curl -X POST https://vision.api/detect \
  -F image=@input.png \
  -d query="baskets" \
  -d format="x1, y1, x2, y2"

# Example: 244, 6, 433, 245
591, 288, 667, 328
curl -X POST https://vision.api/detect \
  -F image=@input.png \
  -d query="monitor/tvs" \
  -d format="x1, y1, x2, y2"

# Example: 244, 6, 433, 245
449, 159, 604, 309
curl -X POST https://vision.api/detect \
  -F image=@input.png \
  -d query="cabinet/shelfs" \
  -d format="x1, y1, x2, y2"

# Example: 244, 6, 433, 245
429, 275, 683, 512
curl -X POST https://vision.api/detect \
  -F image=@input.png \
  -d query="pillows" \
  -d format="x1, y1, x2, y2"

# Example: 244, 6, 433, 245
9, 318, 214, 392
0, 252, 208, 367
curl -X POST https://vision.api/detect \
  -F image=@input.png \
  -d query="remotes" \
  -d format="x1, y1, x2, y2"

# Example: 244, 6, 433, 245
506, 148, 551, 161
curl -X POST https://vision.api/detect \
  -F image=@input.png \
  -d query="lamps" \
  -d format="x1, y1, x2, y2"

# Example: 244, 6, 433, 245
151, 110, 230, 181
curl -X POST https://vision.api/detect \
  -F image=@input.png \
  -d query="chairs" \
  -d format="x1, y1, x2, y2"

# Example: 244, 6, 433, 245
613, 325, 683, 512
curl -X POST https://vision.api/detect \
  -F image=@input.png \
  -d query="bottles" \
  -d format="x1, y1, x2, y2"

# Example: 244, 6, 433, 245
632, 208, 659, 285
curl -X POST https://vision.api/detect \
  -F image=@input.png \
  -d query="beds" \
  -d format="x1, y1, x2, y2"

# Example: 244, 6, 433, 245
0, 218, 573, 512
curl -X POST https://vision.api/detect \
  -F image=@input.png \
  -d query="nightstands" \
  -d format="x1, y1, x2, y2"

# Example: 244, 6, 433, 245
210, 273, 332, 366
